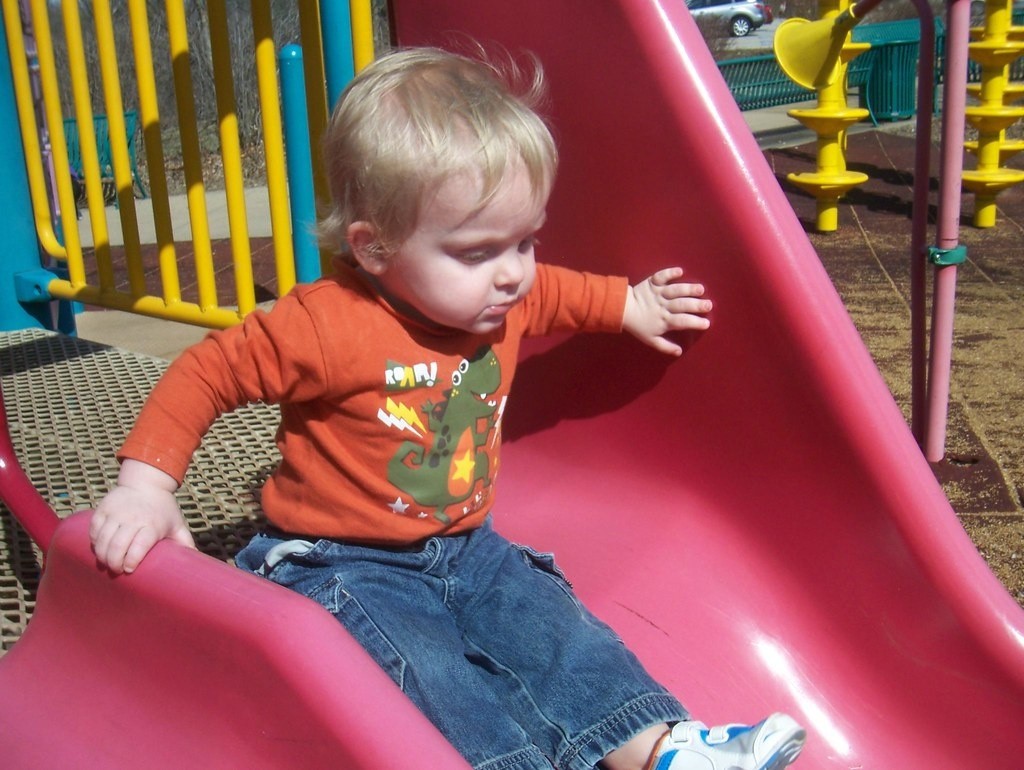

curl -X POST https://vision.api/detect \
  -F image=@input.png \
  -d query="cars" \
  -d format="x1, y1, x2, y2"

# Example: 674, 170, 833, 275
686, 0, 774, 38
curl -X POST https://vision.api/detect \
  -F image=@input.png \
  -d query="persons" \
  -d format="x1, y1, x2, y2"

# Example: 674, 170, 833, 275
89, 45, 810, 770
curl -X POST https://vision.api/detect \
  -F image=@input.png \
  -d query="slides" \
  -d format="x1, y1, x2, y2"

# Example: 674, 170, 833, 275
1, 1, 1024, 770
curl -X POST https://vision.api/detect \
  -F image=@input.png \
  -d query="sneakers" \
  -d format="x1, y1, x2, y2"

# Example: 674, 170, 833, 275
649, 710, 806, 770
